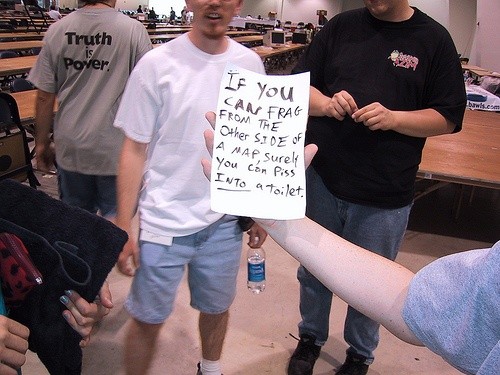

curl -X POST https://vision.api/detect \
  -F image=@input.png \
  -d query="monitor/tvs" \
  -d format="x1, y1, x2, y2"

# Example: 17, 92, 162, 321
263, 31, 286, 50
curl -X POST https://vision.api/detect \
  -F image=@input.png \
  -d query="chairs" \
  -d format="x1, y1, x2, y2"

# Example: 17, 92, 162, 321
11, 77, 33, 91
0, 90, 40, 190
0, 50, 17, 58
29, 47, 41, 55
22, 0, 52, 35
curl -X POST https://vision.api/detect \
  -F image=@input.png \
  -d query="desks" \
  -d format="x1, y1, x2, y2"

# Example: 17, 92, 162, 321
413, 108, 500, 205
0, 10, 311, 125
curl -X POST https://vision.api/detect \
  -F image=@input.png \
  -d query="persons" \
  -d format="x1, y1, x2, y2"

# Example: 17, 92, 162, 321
0, 279, 114, 375
24, 0, 155, 224
49, 5, 189, 23
115, 0, 269, 375
281, 0, 467, 375
198, 113, 500, 375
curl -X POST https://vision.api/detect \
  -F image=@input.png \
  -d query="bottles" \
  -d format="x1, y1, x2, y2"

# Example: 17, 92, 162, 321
247, 236, 266, 295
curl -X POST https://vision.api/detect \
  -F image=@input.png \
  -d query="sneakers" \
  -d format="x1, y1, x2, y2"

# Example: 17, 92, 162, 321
288, 333, 320, 375
335, 356, 368, 375
